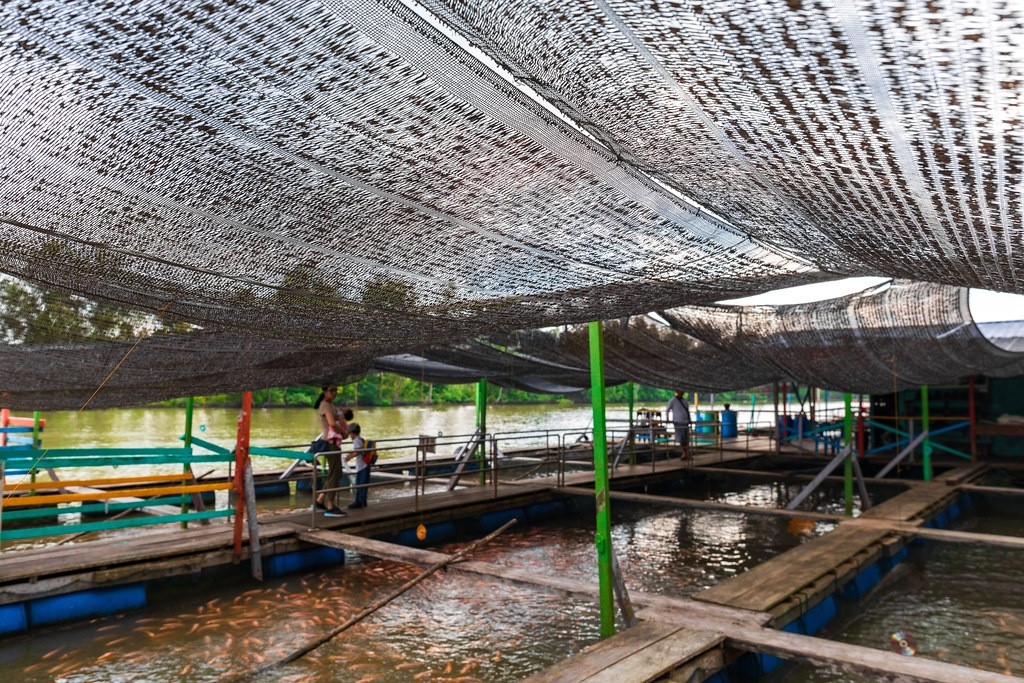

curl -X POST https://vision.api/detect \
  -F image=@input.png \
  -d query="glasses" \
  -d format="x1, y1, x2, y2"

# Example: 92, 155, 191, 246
326, 388, 339, 393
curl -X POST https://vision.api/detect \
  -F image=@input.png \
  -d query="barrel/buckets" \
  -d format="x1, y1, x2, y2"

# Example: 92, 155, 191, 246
721, 411, 737, 438
695, 409, 719, 434
777, 412, 810, 440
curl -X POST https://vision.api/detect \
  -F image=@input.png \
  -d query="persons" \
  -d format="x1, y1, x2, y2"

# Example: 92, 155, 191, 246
325, 408, 354, 446
665, 390, 695, 460
344, 422, 370, 510
314, 384, 348, 518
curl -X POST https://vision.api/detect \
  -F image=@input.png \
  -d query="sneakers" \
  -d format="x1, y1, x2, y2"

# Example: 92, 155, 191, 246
348, 501, 368, 509
314, 501, 347, 517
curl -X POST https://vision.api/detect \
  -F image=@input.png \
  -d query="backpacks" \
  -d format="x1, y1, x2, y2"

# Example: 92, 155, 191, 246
363, 440, 379, 464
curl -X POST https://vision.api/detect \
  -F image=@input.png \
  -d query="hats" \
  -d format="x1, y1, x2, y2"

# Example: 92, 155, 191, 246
347, 422, 360, 432
